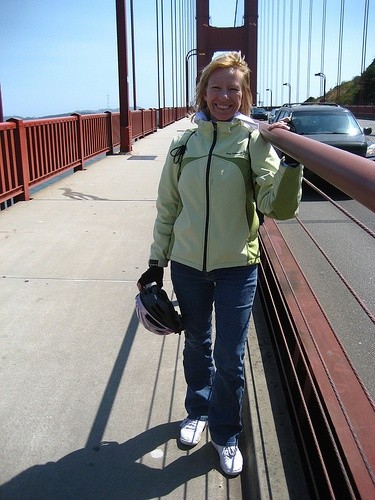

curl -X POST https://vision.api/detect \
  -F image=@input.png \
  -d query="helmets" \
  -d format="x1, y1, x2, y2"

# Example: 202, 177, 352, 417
134, 284, 185, 335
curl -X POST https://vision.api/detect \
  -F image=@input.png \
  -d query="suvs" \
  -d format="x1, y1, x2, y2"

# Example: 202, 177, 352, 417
272, 102, 375, 195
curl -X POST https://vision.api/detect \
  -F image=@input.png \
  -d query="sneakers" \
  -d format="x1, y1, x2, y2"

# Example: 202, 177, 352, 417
210, 437, 243, 475
179, 414, 208, 446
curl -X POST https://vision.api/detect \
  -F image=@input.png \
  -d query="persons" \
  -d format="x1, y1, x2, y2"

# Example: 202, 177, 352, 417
137, 52, 304, 476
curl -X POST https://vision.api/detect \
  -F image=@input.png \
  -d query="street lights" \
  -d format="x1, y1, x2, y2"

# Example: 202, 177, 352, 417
283, 83, 291, 106
266, 89, 273, 111
186, 48, 207, 114
314, 73, 326, 103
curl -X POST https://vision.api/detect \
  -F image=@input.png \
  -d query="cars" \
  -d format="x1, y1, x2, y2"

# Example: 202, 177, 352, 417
268, 108, 281, 124
252, 108, 268, 121
